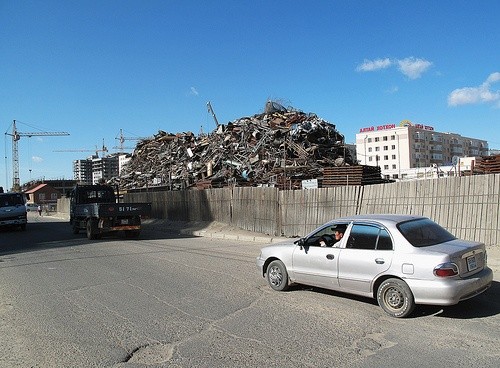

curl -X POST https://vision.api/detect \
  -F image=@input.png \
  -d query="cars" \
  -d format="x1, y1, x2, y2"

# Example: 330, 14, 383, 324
257, 215, 493, 318
25, 202, 41, 212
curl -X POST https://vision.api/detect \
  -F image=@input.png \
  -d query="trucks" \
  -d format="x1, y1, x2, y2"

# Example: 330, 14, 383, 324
0, 192, 31, 233
69, 183, 151, 239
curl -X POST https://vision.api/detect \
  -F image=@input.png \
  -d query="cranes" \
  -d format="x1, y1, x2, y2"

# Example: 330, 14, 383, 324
112, 145, 138, 153
204, 100, 219, 132
115, 128, 149, 144
50, 137, 108, 156
4, 120, 70, 193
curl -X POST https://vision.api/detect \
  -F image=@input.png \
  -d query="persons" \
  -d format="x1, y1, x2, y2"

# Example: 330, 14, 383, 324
319, 224, 348, 248
38, 205, 43, 216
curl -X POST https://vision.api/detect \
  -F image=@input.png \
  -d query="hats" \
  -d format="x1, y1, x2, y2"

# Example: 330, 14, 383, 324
331, 224, 347, 233
